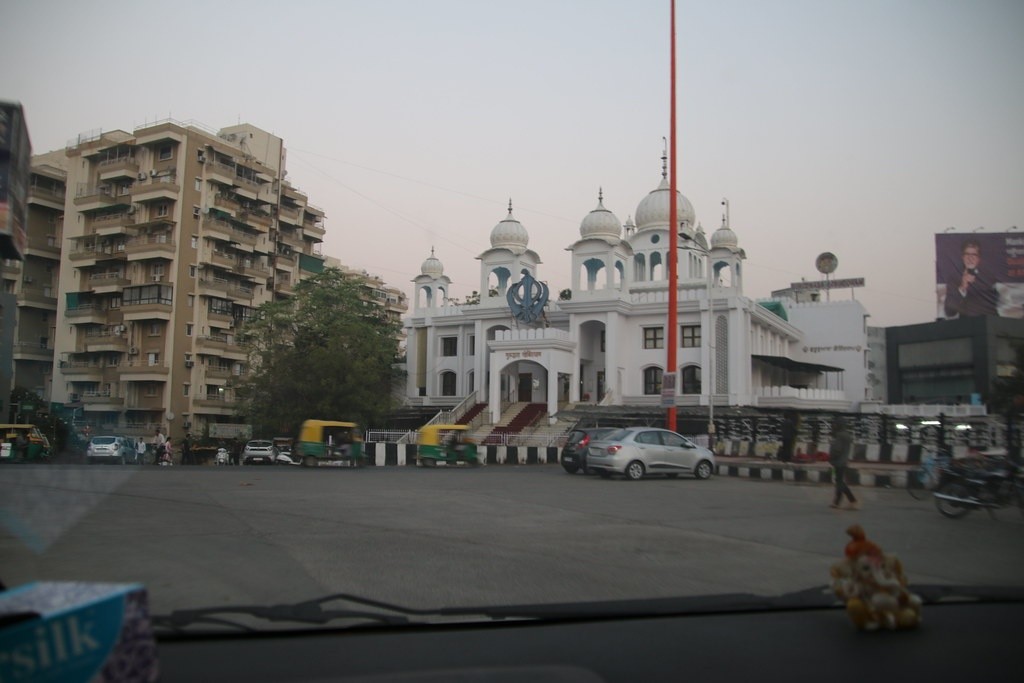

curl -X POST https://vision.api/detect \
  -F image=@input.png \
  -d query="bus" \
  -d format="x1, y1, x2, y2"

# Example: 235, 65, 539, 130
0, 423, 53, 462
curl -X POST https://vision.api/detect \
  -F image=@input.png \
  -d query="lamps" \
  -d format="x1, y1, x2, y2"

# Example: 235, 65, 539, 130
973, 226, 984, 232
1006, 226, 1017, 232
944, 227, 955, 233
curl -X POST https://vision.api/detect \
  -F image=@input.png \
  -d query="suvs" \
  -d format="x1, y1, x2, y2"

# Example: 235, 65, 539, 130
85, 435, 137, 465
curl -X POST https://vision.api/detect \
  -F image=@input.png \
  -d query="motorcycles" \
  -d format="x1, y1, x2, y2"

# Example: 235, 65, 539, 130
411, 423, 478, 467
295, 419, 369, 468
931, 450, 1024, 519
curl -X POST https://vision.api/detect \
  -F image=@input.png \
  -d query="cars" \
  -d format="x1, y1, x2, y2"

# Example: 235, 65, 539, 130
586, 427, 715, 481
560, 427, 629, 474
243, 439, 277, 464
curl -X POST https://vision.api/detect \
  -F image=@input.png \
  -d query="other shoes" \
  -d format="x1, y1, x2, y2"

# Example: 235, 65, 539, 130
847, 500, 859, 509
828, 504, 838, 508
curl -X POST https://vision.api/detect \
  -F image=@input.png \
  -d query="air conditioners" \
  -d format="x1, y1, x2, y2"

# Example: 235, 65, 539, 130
186, 360, 194, 367
152, 274, 160, 281
198, 156, 206, 162
150, 170, 157, 177
60, 360, 70, 368
127, 207, 135, 214
184, 421, 192, 428
23, 275, 32, 281
129, 347, 138, 354
70, 393, 80, 401
137, 173, 146, 180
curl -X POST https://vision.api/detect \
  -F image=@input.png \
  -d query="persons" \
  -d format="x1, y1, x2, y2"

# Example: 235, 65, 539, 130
443, 431, 458, 465
335, 427, 350, 458
828, 418, 858, 509
945, 238, 1003, 320
135, 430, 174, 466
1008, 394, 1024, 421
348, 427, 363, 469
181, 434, 190, 464
216, 437, 243, 465
782, 408, 802, 462
0, 430, 29, 458
